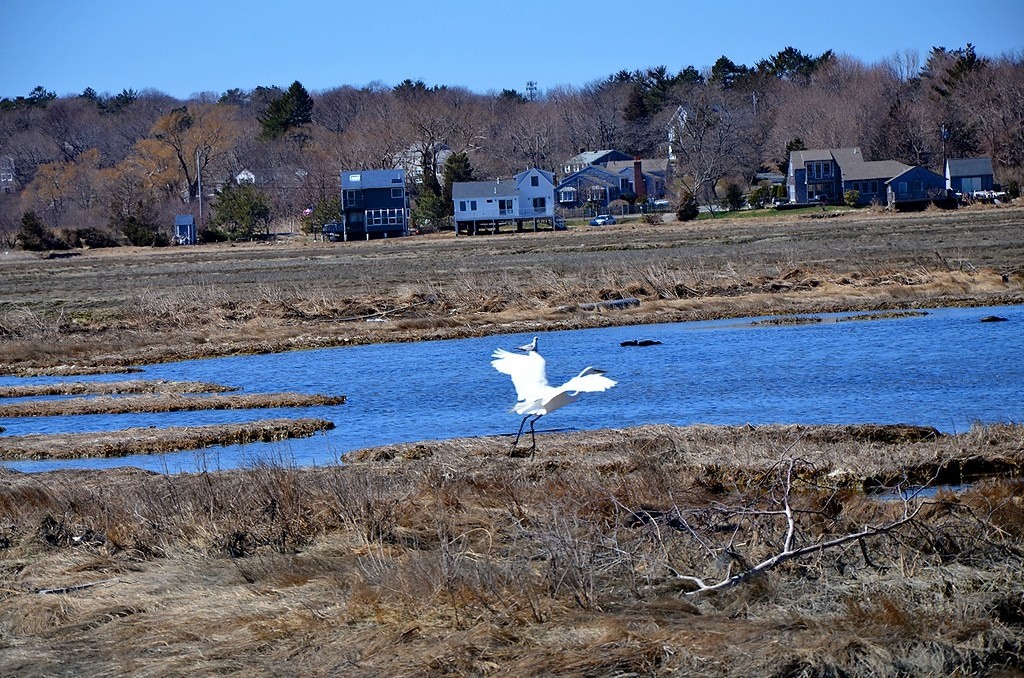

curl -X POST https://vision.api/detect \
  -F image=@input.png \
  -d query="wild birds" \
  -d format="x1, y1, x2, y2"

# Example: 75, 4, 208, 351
491, 347, 618, 462
513, 337, 541, 352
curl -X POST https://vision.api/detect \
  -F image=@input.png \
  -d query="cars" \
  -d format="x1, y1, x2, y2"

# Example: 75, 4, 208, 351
748, 203, 756, 209
972, 189, 1006, 199
588, 214, 617, 226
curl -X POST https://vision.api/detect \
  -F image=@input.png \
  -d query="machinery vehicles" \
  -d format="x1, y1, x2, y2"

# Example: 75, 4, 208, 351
772, 196, 789, 204
